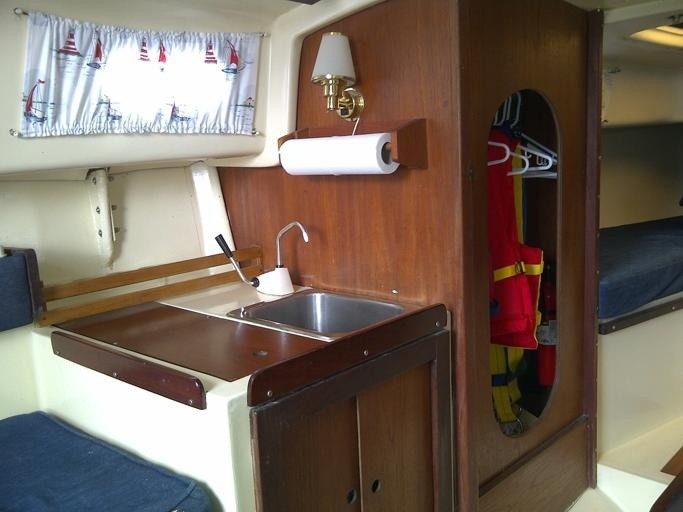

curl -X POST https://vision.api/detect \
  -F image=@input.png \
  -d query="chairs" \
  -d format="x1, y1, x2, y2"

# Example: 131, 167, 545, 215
0, 253, 216, 510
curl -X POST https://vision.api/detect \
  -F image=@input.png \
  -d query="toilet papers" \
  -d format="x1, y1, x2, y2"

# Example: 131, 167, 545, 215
280, 133, 400, 176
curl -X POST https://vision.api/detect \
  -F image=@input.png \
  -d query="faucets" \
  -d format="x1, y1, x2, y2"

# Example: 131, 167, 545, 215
275, 220, 309, 268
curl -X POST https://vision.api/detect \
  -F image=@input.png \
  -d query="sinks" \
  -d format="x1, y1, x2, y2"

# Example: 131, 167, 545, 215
224, 287, 406, 344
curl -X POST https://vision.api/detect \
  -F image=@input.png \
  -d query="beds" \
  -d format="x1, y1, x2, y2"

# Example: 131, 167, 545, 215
597, 217, 681, 452
